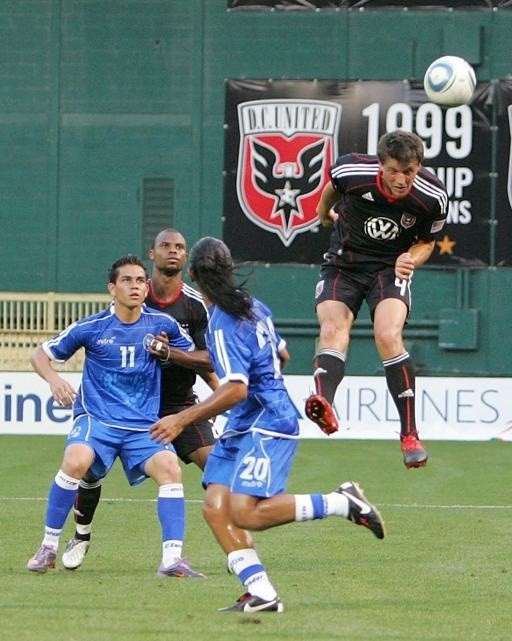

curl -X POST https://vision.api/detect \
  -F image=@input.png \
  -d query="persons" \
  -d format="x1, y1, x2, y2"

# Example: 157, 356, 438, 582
305, 132, 450, 468
62, 227, 217, 573
150, 238, 385, 614
26, 255, 218, 580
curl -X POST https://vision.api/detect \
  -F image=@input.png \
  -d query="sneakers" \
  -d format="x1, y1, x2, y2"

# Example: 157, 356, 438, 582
157, 558, 206, 580
62, 538, 90, 570
26, 544, 56, 573
217, 593, 284, 615
394, 431, 429, 469
334, 482, 385, 541
303, 392, 339, 436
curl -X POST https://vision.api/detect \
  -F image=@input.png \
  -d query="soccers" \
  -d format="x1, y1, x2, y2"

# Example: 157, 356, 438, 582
425, 56, 477, 106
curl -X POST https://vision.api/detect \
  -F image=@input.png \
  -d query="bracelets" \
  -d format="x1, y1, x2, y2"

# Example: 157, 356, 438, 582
160, 346, 170, 362
